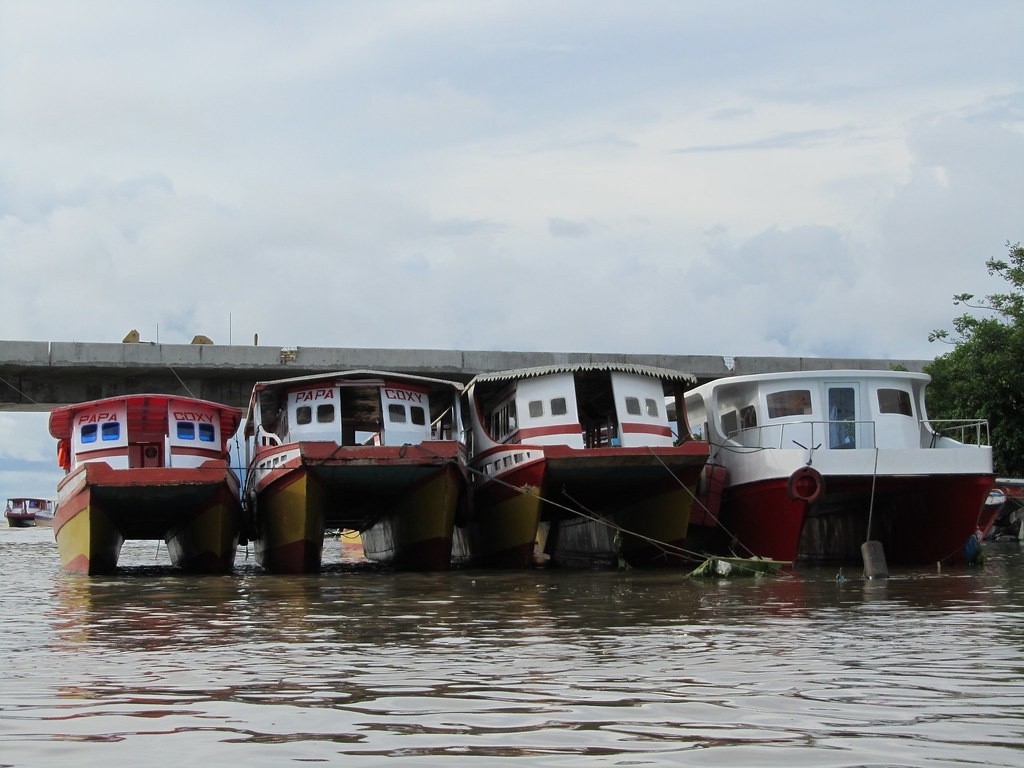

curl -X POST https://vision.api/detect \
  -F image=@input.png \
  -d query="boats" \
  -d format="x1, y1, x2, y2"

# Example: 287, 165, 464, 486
240, 368, 469, 574
664, 368, 997, 564
48, 394, 240, 575
4, 498, 59, 527
452, 362, 710, 567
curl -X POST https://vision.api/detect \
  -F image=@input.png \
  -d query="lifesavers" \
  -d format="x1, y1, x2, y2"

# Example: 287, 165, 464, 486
786, 467, 825, 503
